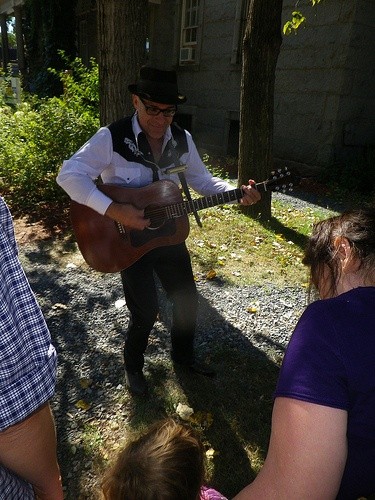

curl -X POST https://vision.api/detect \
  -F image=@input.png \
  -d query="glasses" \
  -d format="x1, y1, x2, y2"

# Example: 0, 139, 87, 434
138, 96, 179, 118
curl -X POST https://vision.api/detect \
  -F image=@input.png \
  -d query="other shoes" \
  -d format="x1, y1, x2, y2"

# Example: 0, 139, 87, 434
124, 370, 148, 395
171, 351, 217, 379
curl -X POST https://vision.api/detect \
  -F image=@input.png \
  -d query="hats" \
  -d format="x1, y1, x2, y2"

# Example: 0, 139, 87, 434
128, 66, 188, 105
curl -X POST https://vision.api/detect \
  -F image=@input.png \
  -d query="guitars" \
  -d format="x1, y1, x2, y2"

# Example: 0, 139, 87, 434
69, 166, 300, 273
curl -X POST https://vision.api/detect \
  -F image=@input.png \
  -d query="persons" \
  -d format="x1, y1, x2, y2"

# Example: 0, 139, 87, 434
0, 194, 63, 500
55, 63, 261, 395
99, 418, 230, 500
231, 209, 375, 500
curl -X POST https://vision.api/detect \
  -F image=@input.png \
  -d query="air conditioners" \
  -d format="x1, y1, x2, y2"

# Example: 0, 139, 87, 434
179, 48, 196, 63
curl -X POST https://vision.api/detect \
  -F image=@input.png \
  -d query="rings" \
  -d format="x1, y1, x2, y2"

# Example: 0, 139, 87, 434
250, 202, 253, 205
